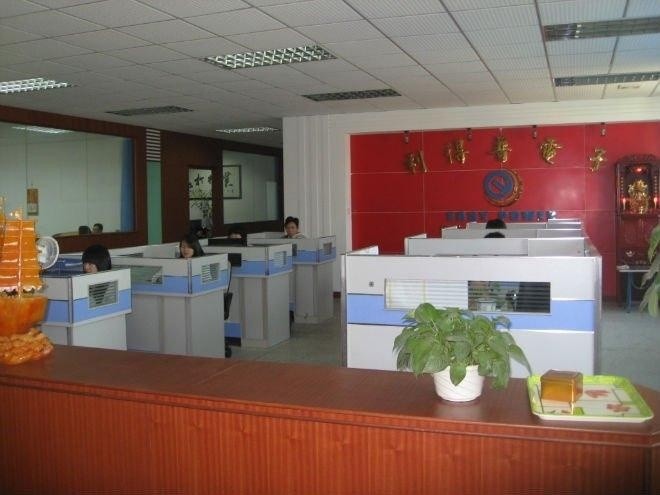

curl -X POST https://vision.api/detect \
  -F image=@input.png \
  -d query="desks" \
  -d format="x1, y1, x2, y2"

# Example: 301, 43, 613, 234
617, 266, 651, 313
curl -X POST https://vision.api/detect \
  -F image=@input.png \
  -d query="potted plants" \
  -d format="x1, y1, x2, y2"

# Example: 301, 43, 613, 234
392, 303, 532, 402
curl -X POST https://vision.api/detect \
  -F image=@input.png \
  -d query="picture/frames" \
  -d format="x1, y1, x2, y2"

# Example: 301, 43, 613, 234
189, 165, 241, 199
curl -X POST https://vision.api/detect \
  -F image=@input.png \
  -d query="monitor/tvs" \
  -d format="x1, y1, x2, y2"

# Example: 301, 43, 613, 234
468, 281, 552, 312
207, 238, 245, 268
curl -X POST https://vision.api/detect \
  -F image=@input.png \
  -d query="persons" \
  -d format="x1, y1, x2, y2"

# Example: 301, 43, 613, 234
485, 232, 504, 238
228, 226, 253, 246
486, 219, 507, 229
93, 223, 104, 233
152, 233, 212, 284
82, 245, 117, 306
280, 216, 305, 238
